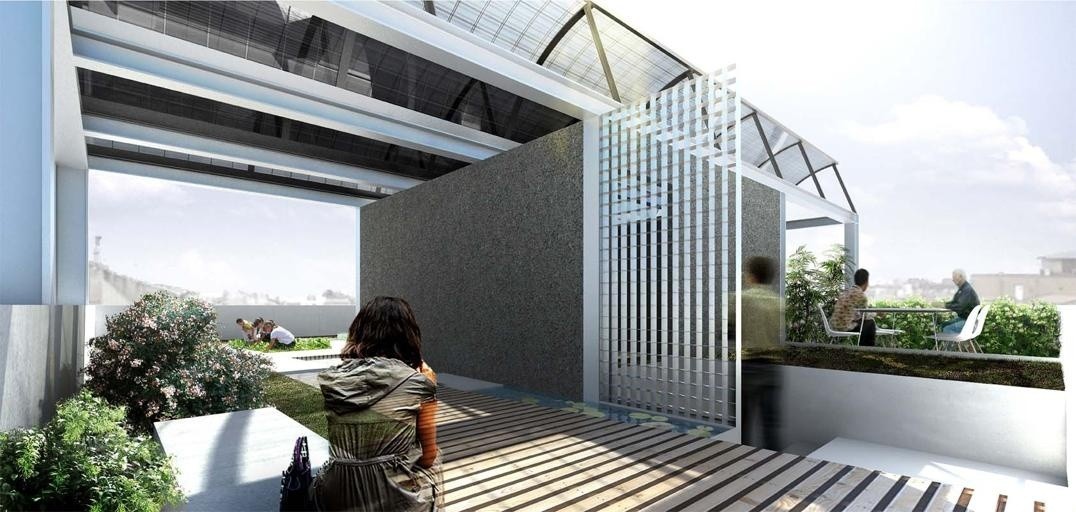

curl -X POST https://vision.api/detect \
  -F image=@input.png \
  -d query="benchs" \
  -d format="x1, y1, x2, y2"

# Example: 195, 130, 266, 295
151, 402, 355, 512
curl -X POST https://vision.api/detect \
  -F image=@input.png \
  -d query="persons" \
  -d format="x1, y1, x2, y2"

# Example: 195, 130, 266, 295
729, 256, 786, 453
236, 317, 296, 349
829, 268, 876, 346
931, 268, 981, 351
310, 297, 445, 511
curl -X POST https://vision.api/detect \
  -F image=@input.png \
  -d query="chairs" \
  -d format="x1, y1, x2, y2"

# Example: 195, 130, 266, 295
816, 302, 990, 354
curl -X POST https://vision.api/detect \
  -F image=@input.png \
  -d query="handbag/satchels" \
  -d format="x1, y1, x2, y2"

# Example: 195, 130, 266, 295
278, 437, 312, 510
